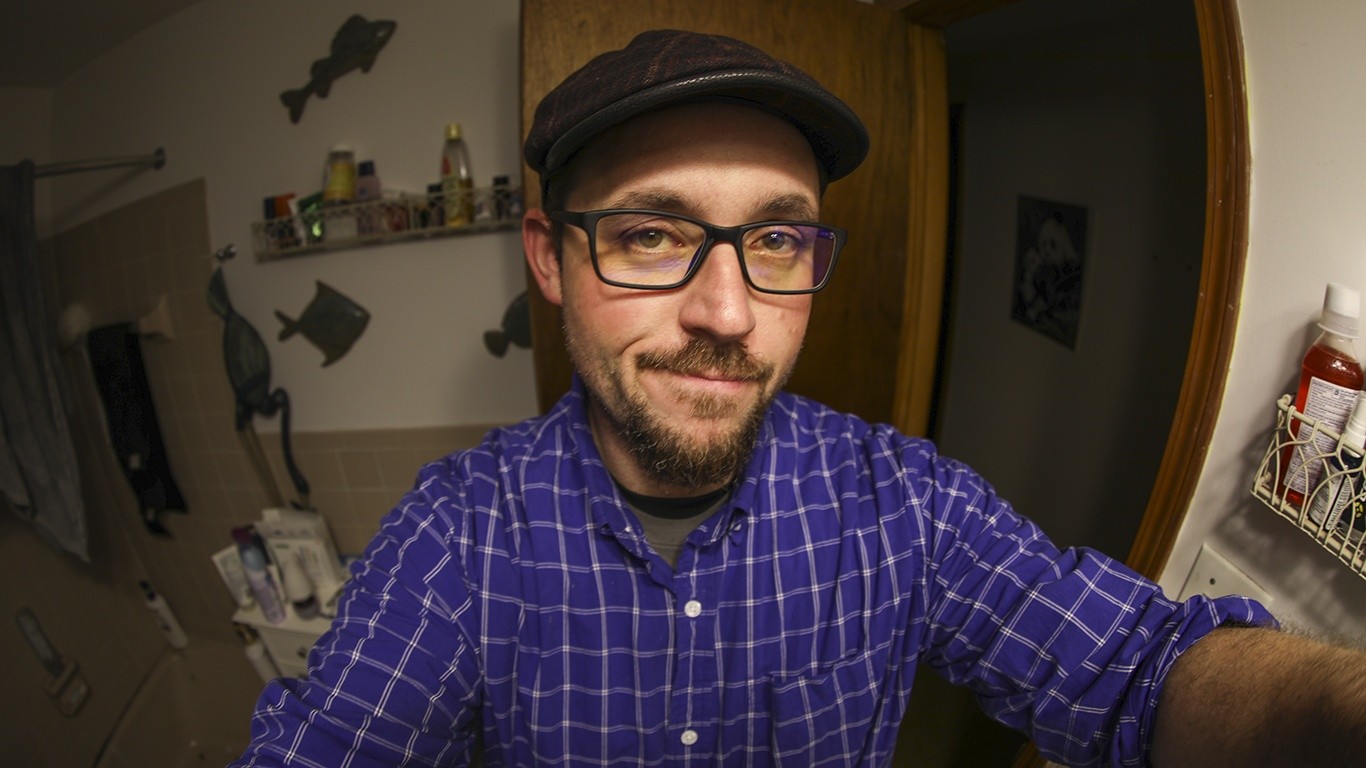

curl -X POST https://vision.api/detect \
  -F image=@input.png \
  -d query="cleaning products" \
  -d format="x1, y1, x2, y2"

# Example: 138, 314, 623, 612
441, 122, 474, 227
1270, 281, 1365, 504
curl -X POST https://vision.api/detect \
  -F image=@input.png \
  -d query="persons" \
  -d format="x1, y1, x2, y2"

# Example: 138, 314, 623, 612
240, 29, 1366, 768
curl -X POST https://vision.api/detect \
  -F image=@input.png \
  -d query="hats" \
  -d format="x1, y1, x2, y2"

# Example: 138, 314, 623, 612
524, 29, 868, 197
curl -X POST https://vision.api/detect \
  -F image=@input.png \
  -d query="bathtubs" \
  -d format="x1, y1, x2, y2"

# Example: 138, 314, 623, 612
91, 637, 265, 768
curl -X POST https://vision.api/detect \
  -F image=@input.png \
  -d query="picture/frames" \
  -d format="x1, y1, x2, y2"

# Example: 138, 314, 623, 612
1009, 192, 1092, 352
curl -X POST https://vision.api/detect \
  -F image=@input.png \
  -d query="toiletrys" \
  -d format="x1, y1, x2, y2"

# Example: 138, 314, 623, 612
233, 623, 280, 685
210, 543, 255, 612
280, 555, 320, 621
242, 549, 287, 625
138, 581, 189, 650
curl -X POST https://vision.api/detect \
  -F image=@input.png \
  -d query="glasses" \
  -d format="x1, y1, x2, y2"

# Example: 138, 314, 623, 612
544, 209, 847, 295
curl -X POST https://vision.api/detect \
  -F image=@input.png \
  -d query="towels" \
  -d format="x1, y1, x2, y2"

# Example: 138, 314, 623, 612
86, 318, 192, 541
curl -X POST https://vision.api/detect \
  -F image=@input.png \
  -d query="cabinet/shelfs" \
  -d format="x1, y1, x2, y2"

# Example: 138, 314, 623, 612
231, 608, 335, 682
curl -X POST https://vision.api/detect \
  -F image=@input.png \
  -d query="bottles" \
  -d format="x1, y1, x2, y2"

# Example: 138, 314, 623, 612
441, 120, 475, 226
321, 144, 357, 208
492, 174, 513, 219
357, 159, 384, 200
1268, 280, 1366, 552
